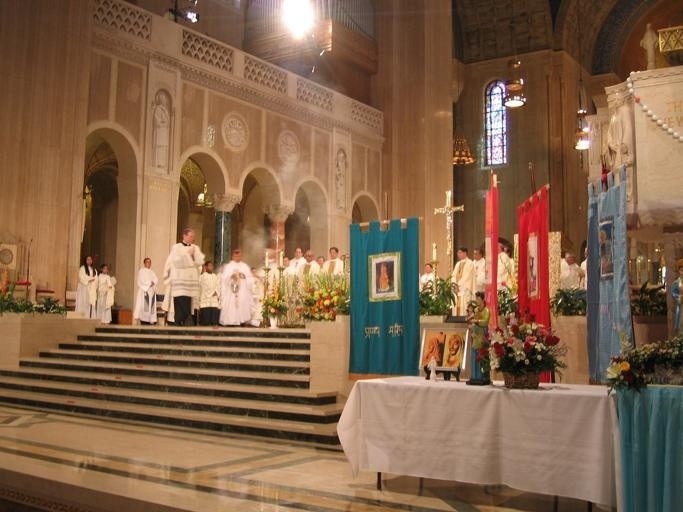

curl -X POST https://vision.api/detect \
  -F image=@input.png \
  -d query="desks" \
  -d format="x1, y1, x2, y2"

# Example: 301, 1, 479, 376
333, 374, 622, 512
607, 385, 682, 511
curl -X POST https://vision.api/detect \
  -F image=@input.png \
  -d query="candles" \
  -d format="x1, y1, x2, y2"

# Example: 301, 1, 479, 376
279, 251, 283, 266
264, 251, 268, 268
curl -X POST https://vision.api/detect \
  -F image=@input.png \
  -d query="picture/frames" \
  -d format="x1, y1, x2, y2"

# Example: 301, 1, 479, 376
416, 325, 469, 370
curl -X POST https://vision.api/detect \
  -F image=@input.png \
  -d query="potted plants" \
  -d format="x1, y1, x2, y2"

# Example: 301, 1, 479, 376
416, 276, 458, 323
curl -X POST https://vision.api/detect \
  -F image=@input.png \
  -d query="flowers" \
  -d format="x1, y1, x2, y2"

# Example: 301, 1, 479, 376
475, 320, 566, 376
605, 335, 682, 392
262, 271, 346, 326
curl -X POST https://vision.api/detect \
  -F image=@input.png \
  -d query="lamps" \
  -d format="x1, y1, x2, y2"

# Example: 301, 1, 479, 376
570, 0, 591, 151
503, 0, 526, 110
447, 0, 473, 166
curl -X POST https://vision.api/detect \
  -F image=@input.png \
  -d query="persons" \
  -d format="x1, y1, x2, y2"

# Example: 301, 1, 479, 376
96, 263, 117, 323
132, 258, 159, 325
450, 240, 517, 315
446, 336, 462, 367
673, 259, 682, 335
160, 229, 206, 326
578, 246, 588, 291
638, 22, 661, 62
199, 244, 343, 325
560, 252, 582, 288
419, 263, 440, 292
73, 255, 97, 319
608, 88, 634, 204
467, 290, 490, 381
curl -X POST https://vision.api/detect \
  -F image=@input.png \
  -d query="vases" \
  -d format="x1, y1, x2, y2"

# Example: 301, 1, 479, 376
500, 370, 540, 388
